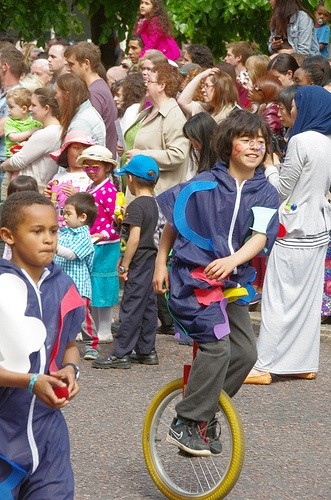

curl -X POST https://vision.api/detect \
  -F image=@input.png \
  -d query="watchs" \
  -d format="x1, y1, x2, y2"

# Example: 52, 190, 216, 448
118, 266, 129, 273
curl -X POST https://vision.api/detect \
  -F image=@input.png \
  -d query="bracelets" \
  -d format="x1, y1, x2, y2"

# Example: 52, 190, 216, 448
28, 373, 39, 394
62, 363, 79, 381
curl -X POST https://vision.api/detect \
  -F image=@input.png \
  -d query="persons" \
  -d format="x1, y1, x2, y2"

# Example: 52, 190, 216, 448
60, 146, 121, 342
0, 191, 86, 500
152, 109, 280, 457
243, 85, 331, 385
91, 155, 159, 369
53, 192, 101, 359
0, 0, 330, 334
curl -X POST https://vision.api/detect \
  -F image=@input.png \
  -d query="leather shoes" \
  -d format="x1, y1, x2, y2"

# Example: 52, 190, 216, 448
129, 350, 159, 365
91, 352, 131, 369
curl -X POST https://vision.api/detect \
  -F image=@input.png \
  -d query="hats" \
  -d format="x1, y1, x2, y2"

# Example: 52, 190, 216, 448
48, 130, 96, 161
114, 153, 160, 180
106, 64, 129, 84
75, 145, 118, 168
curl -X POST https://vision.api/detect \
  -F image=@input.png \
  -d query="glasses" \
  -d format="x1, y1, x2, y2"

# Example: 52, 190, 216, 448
82, 164, 103, 174
148, 81, 159, 85
253, 83, 265, 100
201, 83, 214, 88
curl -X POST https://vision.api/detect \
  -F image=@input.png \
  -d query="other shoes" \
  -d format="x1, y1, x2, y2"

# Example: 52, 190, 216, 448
276, 373, 318, 380
247, 290, 263, 306
156, 328, 176, 335
243, 372, 272, 385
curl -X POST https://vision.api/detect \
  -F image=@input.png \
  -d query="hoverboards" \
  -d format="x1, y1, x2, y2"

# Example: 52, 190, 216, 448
141, 286, 250, 500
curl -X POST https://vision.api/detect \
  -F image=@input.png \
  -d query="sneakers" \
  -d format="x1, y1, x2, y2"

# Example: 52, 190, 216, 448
205, 414, 223, 456
165, 417, 212, 456
83, 348, 101, 359
